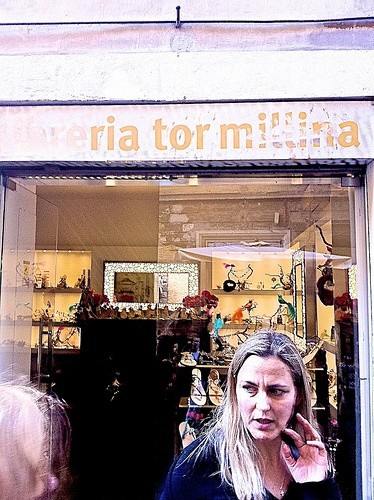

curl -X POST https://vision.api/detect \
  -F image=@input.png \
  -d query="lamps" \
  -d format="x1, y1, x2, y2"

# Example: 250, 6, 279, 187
105, 175, 116, 187
188, 174, 199, 187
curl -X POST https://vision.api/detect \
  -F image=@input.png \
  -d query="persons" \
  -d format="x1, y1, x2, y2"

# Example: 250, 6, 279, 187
1, 382, 72, 500
160, 330, 343, 500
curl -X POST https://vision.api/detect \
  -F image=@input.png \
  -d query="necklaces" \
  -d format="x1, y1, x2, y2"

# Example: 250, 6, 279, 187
261, 468, 295, 498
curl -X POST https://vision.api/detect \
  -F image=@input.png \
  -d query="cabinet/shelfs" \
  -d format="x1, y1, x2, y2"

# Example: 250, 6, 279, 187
176, 348, 329, 467
204, 234, 296, 355
0, 249, 92, 354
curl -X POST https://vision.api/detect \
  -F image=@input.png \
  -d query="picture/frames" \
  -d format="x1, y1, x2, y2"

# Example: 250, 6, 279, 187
291, 245, 307, 353
102, 260, 201, 313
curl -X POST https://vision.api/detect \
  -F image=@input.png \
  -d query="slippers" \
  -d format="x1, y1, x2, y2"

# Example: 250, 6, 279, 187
180, 350, 197, 366
178, 421, 197, 450
190, 368, 207, 406
207, 369, 226, 406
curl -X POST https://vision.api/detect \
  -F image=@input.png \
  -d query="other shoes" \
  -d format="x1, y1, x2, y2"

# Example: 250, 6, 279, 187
99, 304, 197, 319
